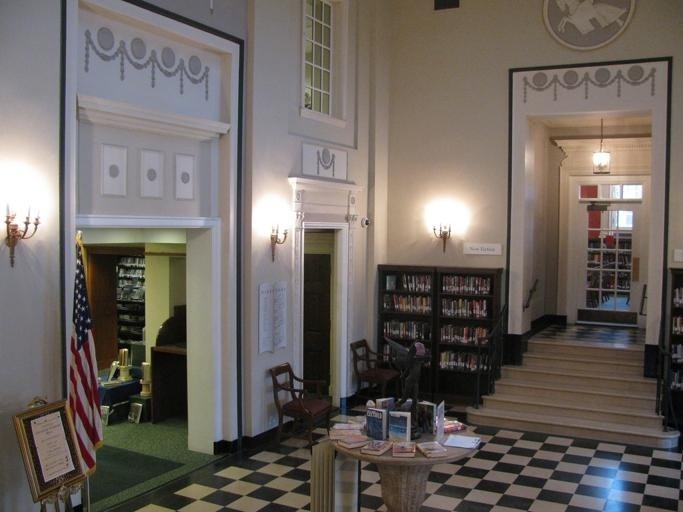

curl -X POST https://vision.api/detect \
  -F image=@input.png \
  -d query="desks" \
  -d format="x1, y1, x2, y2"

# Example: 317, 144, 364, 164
327, 421, 481, 512
150, 340, 187, 424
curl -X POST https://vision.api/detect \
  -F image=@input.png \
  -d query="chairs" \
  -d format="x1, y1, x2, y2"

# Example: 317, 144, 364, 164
270, 362, 335, 449
586, 262, 610, 307
351, 338, 402, 406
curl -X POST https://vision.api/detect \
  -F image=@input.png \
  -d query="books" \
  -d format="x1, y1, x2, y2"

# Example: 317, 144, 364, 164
670, 285, 683, 391
587, 233, 631, 309
325, 395, 483, 459
116, 257, 144, 334
382, 274, 490, 370
126, 402, 143, 425
99, 404, 109, 426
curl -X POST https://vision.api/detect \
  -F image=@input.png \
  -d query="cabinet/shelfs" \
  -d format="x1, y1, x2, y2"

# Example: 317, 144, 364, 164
113, 255, 145, 353
588, 235, 633, 294
660, 266, 683, 426
378, 264, 505, 409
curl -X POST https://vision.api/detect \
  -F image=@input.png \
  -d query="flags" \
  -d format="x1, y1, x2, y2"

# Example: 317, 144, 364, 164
64, 227, 104, 481
